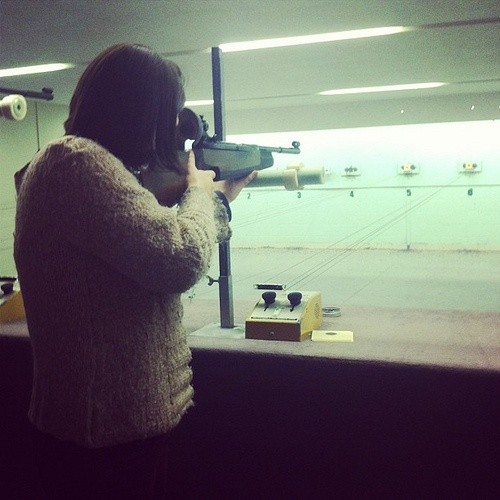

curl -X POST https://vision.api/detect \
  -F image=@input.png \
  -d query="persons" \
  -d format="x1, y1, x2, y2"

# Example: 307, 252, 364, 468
10, 42, 258, 500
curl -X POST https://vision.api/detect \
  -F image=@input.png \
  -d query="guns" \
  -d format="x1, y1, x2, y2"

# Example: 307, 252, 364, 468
175, 107, 302, 182
0, 84, 54, 102
